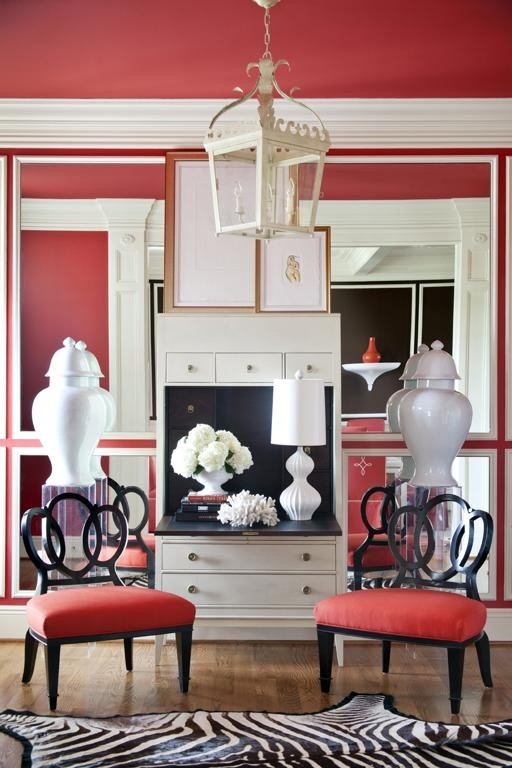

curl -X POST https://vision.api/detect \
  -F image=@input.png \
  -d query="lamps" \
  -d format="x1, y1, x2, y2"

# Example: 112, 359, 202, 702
269, 370, 329, 522
199, 1, 330, 242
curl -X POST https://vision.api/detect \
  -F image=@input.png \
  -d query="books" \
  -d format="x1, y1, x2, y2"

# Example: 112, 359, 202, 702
174, 491, 236, 522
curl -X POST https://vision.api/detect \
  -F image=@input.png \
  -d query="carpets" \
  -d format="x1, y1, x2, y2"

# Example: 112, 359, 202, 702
1, 687, 511, 767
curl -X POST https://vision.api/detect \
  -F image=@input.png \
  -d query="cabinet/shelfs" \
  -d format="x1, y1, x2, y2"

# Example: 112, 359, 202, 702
157, 315, 341, 386
153, 532, 348, 673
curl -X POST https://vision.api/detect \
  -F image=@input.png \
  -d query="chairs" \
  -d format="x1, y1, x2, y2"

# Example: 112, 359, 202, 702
313, 493, 495, 718
19, 495, 194, 714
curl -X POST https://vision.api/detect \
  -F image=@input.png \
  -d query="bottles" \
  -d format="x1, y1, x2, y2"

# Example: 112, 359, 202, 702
362, 336, 382, 362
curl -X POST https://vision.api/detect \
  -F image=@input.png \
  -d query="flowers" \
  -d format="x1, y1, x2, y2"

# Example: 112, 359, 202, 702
216, 487, 281, 530
171, 421, 256, 479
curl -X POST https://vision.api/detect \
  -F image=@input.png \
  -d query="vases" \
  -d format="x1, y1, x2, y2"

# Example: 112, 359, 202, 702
191, 471, 235, 498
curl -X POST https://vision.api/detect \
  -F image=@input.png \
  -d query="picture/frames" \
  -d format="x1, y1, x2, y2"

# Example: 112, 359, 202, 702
162, 150, 333, 316
